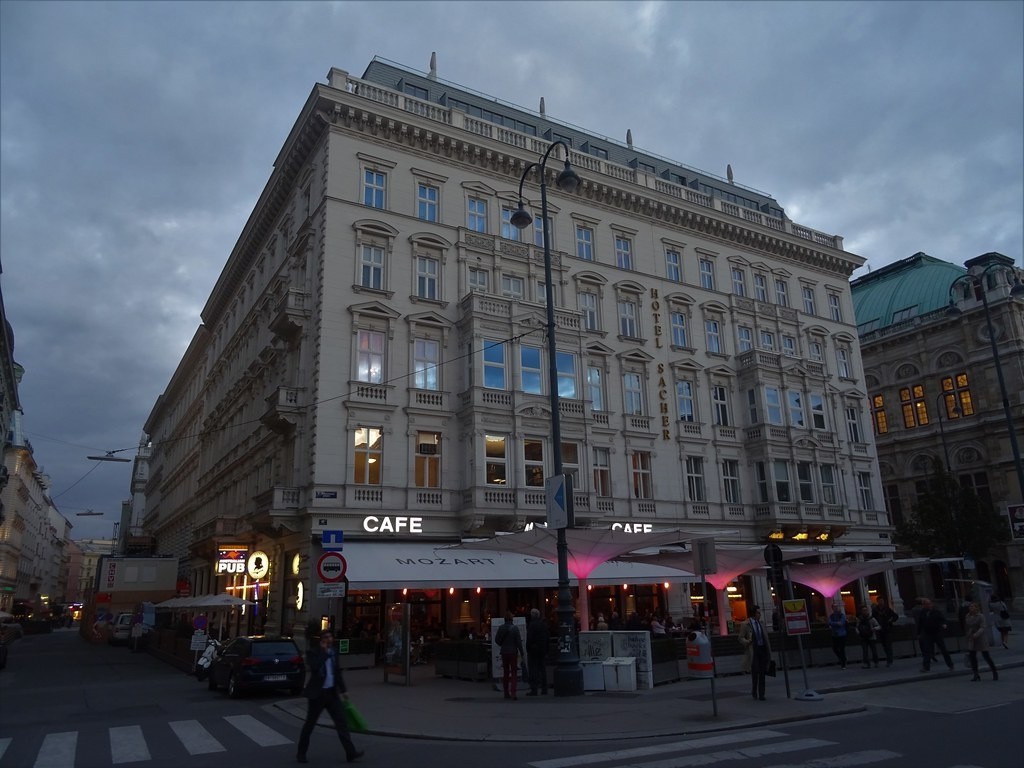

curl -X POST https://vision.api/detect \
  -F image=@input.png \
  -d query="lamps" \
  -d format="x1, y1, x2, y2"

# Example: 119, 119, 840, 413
369, 457, 377, 464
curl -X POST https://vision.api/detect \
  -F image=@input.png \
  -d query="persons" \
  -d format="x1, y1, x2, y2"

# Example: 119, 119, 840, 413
296, 629, 365, 762
738, 606, 770, 700
372, 606, 707, 702
771, 593, 1010, 682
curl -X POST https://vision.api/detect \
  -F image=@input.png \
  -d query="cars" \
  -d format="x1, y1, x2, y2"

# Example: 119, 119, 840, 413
108, 613, 133, 645
209, 635, 306, 696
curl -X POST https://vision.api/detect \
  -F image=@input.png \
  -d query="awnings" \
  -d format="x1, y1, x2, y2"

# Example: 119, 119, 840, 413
329, 541, 702, 589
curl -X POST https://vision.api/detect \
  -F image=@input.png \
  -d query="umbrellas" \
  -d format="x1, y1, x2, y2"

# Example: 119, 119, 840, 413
148, 592, 257, 642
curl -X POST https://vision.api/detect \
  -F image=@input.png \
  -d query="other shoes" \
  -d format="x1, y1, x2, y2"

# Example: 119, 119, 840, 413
753, 692, 758, 700
541, 691, 547, 694
839, 658, 955, 672
759, 695, 766, 701
512, 697, 517, 700
1002, 642, 1008, 649
504, 695, 511, 698
296, 752, 308, 763
526, 691, 537, 696
347, 749, 365, 762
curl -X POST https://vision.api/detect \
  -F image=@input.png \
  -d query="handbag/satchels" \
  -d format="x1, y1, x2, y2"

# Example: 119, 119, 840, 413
340, 695, 369, 730
1000, 601, 1009, 620
520, 660, 529, 680
766, 660, 776, 677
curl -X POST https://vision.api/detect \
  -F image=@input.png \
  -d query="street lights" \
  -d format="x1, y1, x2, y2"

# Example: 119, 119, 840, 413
936, 392, 968, 586
511, 141, 583, 698
944, 261, 1024, 502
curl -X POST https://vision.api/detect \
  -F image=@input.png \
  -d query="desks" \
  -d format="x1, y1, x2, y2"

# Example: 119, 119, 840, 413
412, 642, 432, 665
670, 628, 694, 637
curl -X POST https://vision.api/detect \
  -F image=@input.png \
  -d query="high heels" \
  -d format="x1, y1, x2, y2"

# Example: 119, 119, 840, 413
992, 671, 998, 680
971, 674, 980, 681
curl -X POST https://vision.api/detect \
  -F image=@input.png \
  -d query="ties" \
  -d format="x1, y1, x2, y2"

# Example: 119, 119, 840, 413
755, 620, 761, 641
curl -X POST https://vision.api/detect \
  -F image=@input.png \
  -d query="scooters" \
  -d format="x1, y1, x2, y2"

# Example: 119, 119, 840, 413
196, 637, 231, 678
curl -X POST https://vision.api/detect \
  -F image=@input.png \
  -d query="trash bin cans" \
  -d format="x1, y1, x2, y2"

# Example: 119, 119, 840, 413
686, 632, 713, 679
